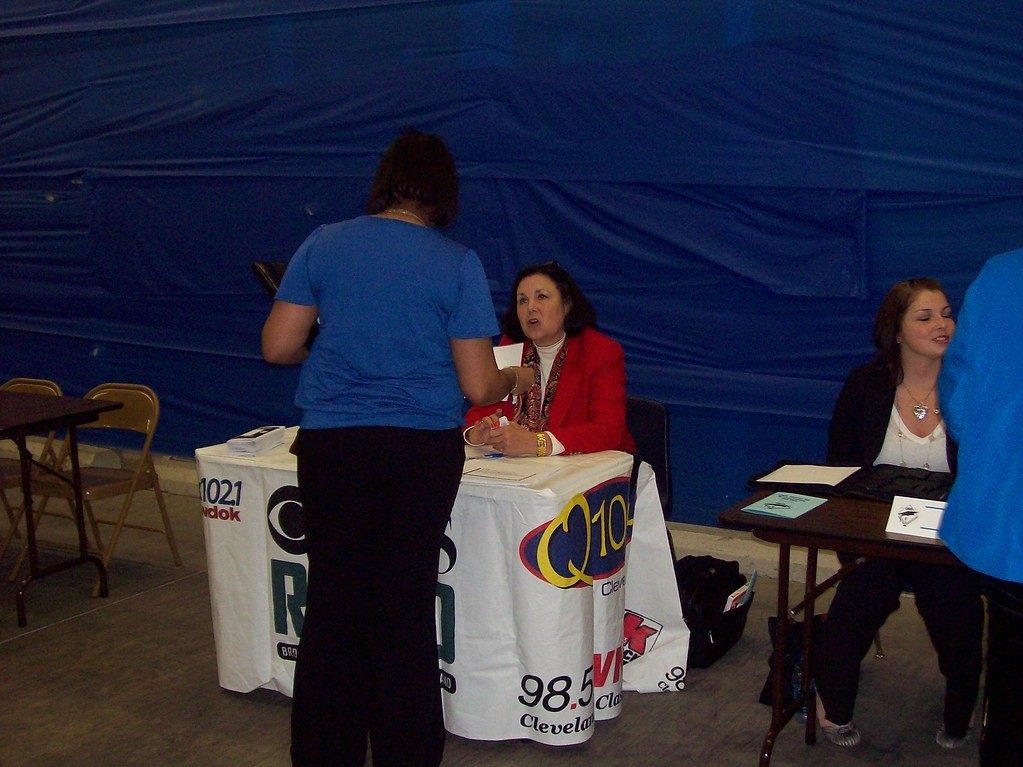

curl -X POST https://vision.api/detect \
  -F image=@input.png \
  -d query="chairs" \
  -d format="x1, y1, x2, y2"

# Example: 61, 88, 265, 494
0, 378, 183, 597
625, 398, 683, 593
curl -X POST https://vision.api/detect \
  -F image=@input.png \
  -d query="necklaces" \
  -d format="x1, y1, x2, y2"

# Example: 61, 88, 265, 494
383, 206, 423, 224
901, 381, 937, 419
895, 379, 940, 470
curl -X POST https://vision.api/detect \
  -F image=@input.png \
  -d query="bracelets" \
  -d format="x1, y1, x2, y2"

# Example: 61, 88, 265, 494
504, 367, 518, 393
536, 433, 547, 456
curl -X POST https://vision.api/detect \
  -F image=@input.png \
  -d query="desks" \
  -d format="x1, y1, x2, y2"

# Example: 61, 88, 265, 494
718, 487, 990, 767
195, 424, 691, 747
1, 388, 125, 628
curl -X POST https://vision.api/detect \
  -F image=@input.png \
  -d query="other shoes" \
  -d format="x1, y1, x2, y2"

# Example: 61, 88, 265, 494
936, 715, 975, 748
814, 693, 862, 747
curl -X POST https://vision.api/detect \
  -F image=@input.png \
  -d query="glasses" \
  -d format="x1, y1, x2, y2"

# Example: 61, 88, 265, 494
527, 259, 559, 271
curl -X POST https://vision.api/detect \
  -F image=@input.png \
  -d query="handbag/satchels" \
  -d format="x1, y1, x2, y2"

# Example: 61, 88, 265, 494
760, 612, 826, 714
670, 554, 754, 667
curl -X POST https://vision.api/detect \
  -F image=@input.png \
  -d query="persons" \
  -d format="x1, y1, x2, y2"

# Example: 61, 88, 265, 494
812, 247, 1023, 767
252, 128, 535, 767
462, 259, 637, 457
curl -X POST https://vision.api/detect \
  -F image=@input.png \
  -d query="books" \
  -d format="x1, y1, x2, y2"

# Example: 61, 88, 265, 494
740, 492, 828, 519
723, 582, 750, 613
884, 496, 950, 540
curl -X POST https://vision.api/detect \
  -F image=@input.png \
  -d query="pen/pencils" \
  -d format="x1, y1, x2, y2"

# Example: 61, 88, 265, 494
469, 453, 506, 459
